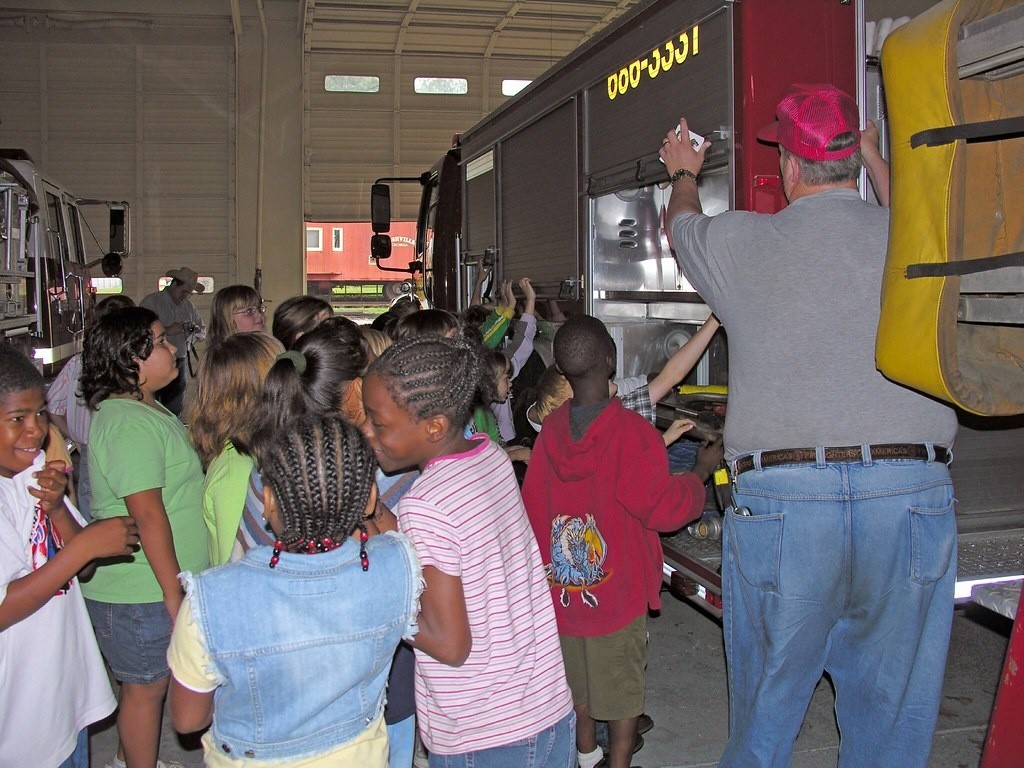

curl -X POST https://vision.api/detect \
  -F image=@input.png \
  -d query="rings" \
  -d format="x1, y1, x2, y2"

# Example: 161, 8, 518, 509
663, 141, 670, 145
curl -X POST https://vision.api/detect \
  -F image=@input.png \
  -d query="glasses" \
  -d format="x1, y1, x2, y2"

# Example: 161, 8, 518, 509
235, 305, 267, 316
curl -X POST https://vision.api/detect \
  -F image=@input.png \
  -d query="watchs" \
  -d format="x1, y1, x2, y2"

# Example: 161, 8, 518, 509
671, 169, 697, 188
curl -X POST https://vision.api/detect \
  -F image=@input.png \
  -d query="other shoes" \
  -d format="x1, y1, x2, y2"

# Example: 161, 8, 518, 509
578, 756, 643, 768
636, 713, 654, 734
603, 733, 644, 754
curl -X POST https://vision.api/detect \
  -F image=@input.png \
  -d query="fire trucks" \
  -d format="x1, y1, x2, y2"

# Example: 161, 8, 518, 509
369, 1, 1024, 620
1, 146, 128, 414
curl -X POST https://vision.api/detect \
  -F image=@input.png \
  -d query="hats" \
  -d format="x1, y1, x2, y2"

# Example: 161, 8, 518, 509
166, 267, 205, 293
757, 84, 861, 161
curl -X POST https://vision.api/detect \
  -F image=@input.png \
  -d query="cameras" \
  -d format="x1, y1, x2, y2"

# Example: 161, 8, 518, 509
184, 321, 196, 333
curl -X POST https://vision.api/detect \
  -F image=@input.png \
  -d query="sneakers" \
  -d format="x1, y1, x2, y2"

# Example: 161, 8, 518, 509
414, 727, 429, 768
104, 754, 185, 768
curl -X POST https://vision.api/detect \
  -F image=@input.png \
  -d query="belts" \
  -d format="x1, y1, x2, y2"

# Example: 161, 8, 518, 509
737, 444, 951, 475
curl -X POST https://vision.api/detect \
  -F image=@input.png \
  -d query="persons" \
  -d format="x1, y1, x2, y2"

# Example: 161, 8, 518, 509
166, 278, 723, 768
45, 294, 136, 522
659, 84, 958, 768
0, 356, 140, 768
140, 267, 207, 419
79, 306, 208, 768
